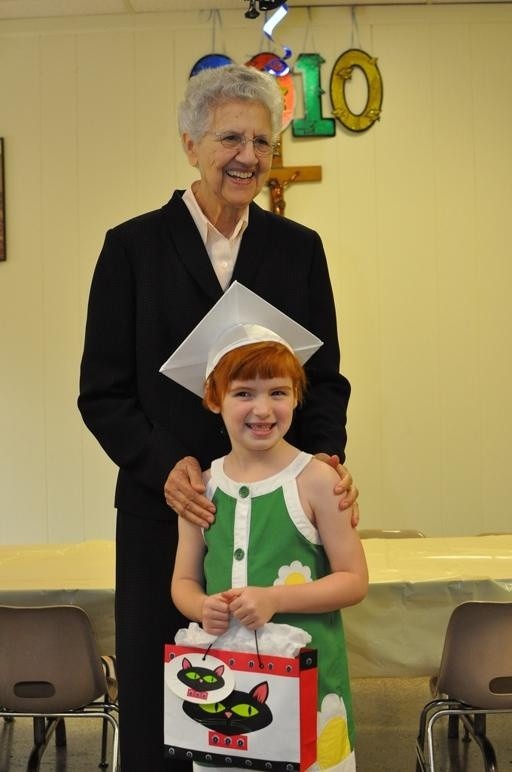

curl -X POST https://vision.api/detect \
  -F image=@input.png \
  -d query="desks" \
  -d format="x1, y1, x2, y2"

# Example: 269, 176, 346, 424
0, 535, 512, 772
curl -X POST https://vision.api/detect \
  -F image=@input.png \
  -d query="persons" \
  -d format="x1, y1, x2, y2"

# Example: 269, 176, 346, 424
77, 62, 363, 772
170, 323, 373, 772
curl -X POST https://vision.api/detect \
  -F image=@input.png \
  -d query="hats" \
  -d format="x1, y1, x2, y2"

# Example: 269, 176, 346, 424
158, 279, 325, 399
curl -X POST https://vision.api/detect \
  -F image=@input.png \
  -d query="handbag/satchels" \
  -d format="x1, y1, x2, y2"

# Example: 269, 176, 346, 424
163, 642, 318, 772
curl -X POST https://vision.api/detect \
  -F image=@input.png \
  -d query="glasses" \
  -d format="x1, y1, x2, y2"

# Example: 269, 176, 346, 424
206, 131, 277, 154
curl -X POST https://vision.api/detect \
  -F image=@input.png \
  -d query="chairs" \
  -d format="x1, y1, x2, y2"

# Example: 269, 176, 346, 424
2, 605, 122, 771
416, 600, 512, 772
